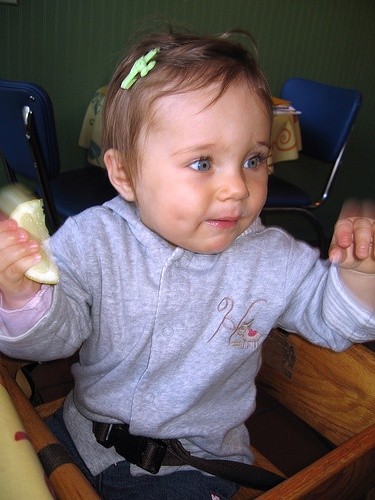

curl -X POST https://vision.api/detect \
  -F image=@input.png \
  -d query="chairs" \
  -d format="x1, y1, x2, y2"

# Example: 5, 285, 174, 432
0, 79, 374, 500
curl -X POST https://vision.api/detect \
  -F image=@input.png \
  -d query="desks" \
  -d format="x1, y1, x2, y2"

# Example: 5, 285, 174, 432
78, 86, 303, 169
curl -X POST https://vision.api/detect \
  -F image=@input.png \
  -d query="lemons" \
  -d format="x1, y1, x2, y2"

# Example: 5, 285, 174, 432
9, 197, 59, 285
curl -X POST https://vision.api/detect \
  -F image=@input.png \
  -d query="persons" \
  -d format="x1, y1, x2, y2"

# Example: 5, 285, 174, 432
1, 33, 375, 500
